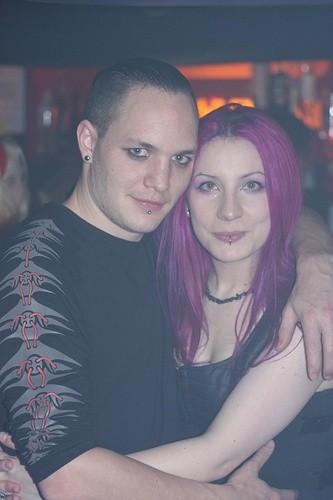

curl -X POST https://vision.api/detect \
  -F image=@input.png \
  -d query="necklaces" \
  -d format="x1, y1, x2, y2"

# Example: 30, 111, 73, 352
202, 285, 254, 304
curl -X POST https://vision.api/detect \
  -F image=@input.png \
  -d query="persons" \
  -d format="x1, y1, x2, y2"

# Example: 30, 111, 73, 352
1, 59, 333, 500
0, 103, 333, 500
